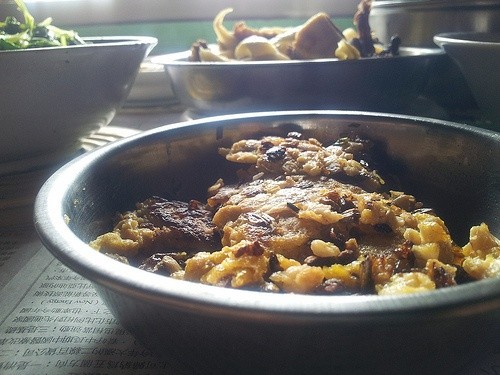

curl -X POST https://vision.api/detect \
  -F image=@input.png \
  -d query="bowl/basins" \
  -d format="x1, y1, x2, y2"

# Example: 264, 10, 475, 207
34, 110, 500, 359
433, 32, 500, 114
0, 36, 158, 172
150, 46, 449, 120
127, 61, 174, 100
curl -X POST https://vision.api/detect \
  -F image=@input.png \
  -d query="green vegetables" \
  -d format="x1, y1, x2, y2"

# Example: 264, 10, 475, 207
0, 0, 89, 51
